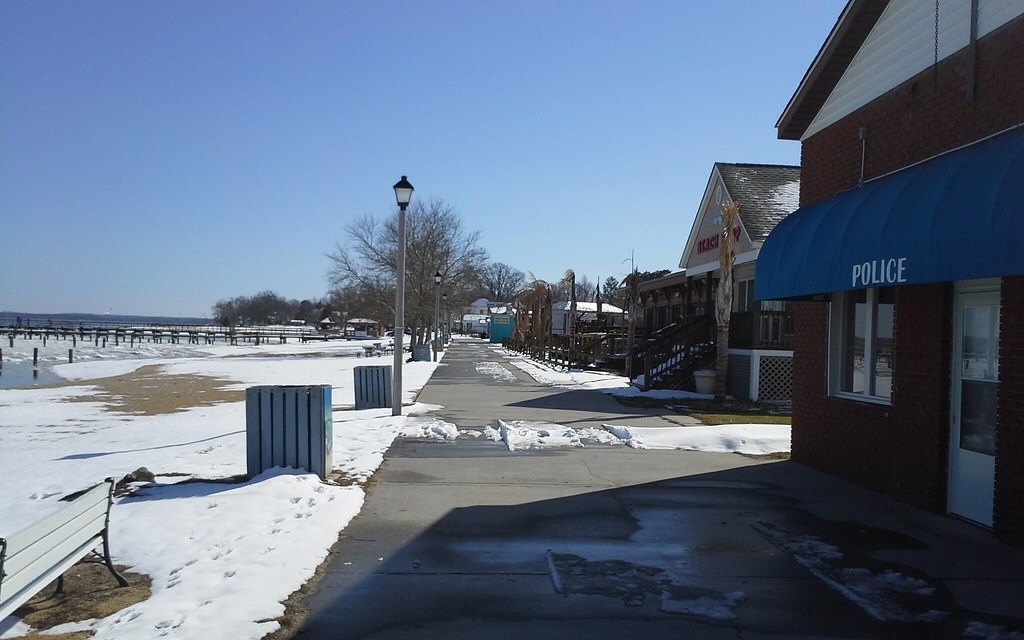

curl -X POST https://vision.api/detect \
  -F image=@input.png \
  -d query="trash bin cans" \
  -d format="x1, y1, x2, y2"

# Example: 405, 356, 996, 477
431, 339, 443, 351
692, 370, 716, 395
414, 344, 431, 362
353, 365, 394, 410
244, 384, 333, 479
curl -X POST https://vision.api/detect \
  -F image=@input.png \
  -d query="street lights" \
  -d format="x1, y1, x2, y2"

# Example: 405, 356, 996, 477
443, 291, 454, 344
434, 268, 443, 362
392, 176, 415, 417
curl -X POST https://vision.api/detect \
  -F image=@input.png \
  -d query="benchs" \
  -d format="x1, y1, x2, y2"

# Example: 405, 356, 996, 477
359, 342, 394, 358
0, 477, 128, 622
